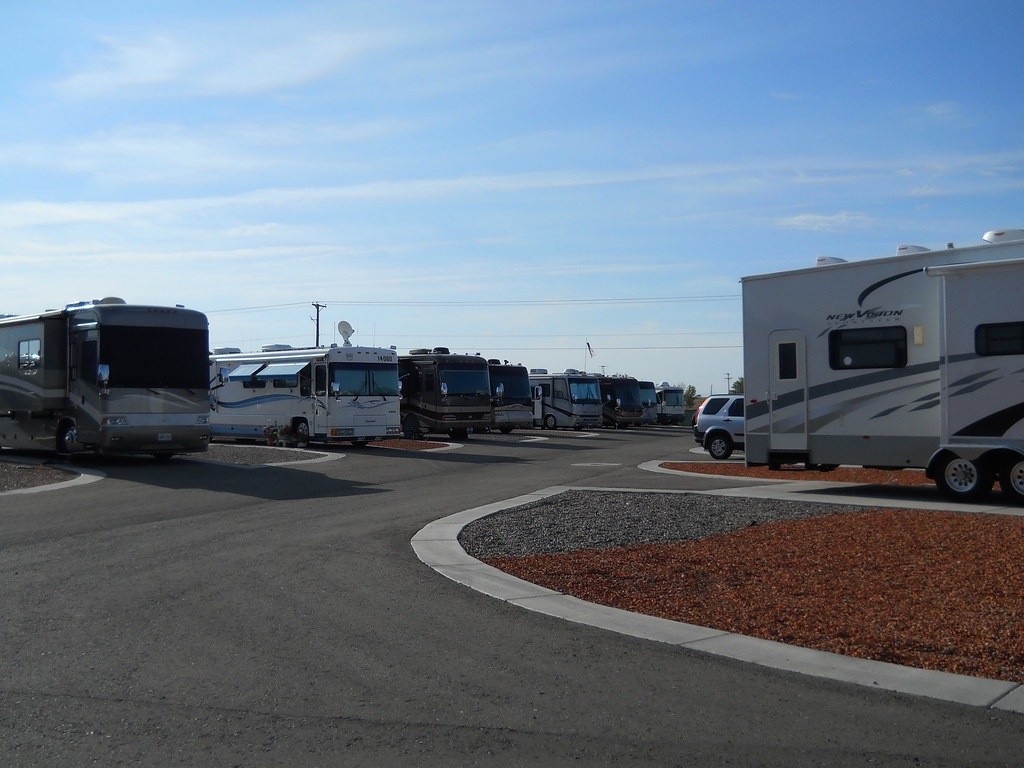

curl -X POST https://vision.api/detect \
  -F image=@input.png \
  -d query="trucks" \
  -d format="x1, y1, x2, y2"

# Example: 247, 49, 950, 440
742, 230, 1023, 474
923, 258, 1024, 508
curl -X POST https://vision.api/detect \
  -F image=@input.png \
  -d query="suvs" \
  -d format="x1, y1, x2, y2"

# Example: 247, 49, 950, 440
691, 393, 744, 460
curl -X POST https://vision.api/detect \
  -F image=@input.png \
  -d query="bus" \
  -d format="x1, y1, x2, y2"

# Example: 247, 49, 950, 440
397, 346, 493, 440
598, 373, 644, 430
208, 344, 404, 448
637, 380, 658, 425
0, 296, 230, 466
655, 381, 686, 426
486, 358, 534, 434
527, 368, 605, 432
